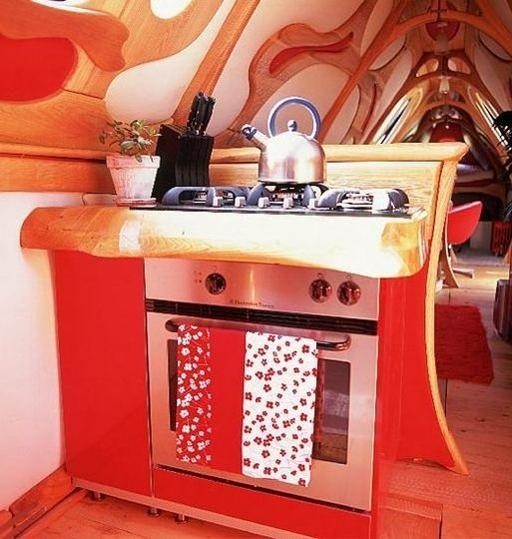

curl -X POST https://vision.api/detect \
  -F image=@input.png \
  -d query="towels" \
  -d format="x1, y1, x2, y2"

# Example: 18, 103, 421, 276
241, 330, 319, 489
175, 323, 247, 474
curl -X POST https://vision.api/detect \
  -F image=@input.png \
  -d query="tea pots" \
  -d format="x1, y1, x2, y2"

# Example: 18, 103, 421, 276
240, 94, 325, 187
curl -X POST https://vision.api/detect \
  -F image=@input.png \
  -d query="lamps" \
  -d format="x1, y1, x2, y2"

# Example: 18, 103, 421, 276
427, 3, 453, 60
437, 56, 453, 95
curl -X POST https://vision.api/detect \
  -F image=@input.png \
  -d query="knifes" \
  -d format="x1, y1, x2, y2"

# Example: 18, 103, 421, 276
187, 90, 215, 135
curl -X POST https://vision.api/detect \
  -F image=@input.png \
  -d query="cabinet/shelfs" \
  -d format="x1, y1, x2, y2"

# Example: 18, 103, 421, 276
137, 312, 379, 522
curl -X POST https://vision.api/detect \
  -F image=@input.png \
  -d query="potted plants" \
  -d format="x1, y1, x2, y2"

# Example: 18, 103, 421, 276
100, 115, 161, 203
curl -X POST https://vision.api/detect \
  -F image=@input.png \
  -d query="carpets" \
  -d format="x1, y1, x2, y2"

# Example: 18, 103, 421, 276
430, 302, 495, 386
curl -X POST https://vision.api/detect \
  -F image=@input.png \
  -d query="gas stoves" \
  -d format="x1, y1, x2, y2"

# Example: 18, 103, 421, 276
128, 179, 425, 221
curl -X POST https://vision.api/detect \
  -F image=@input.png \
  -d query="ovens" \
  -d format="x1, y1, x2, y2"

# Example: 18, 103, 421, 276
141, 256, 380, 513
